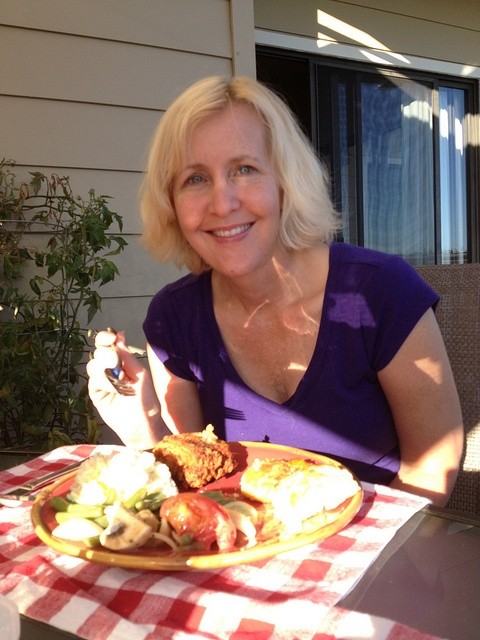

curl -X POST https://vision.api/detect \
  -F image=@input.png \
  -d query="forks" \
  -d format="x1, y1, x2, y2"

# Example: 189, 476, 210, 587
104, 327, 136, 396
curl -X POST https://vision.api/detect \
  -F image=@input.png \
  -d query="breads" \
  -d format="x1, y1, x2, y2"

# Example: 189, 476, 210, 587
239, 453, 363, 535
151, 429, 238, 491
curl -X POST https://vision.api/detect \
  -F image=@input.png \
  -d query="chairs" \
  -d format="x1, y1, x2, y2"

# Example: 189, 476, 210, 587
413, 264, 480, 520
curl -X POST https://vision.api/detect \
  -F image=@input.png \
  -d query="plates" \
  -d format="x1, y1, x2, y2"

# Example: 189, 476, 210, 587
30, 441, 365, 571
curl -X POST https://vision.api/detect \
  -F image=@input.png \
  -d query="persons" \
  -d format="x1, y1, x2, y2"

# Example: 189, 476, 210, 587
86, 75, 465, 510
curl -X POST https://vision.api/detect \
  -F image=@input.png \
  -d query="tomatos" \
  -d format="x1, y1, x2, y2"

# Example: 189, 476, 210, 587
157, 494, 237, 551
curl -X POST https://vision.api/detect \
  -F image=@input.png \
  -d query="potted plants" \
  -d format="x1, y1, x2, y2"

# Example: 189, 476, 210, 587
0, 158, 127, 468
